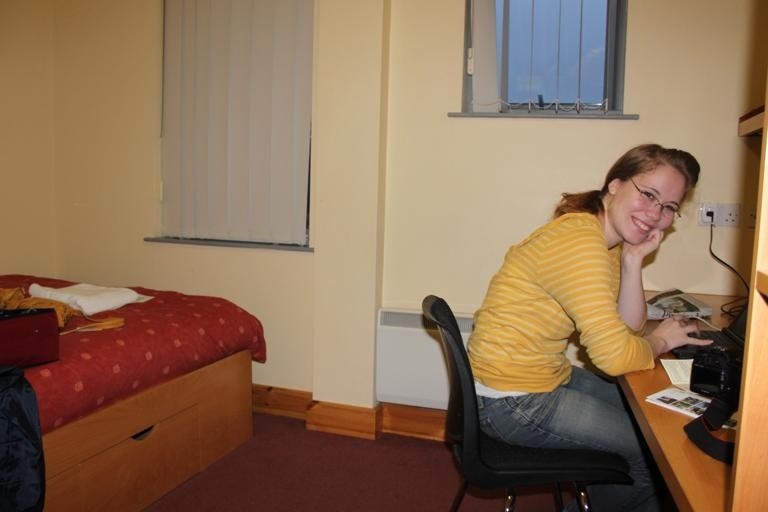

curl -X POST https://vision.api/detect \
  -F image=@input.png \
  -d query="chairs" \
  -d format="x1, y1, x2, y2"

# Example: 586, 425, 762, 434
420, 293, 637, 511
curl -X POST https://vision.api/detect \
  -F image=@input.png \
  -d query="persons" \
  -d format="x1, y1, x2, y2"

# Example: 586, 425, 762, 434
466, 143, 715, 512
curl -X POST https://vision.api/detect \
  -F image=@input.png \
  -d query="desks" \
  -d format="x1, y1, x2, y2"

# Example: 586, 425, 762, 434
613, 290, 767, 511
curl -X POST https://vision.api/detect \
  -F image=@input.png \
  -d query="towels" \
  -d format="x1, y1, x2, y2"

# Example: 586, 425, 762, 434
28, 283, 156, 317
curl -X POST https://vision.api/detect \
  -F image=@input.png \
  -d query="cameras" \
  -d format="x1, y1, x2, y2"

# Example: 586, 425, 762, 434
690, 354, 743, 399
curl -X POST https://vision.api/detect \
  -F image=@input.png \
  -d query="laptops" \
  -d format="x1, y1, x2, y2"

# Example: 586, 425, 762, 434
671, 301, 748, 359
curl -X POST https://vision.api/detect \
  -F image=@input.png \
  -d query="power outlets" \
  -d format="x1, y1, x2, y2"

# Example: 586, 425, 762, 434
697, 203, 758, 229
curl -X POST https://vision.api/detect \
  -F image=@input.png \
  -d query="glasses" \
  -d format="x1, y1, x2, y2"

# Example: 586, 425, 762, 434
629, 176, 681, 220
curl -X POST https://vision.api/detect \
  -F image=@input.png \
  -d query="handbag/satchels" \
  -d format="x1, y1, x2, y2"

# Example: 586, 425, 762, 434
1, 308, 60, 368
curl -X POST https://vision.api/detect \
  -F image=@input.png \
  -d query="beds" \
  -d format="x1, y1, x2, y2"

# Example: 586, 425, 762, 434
1, 273, 254, 512
